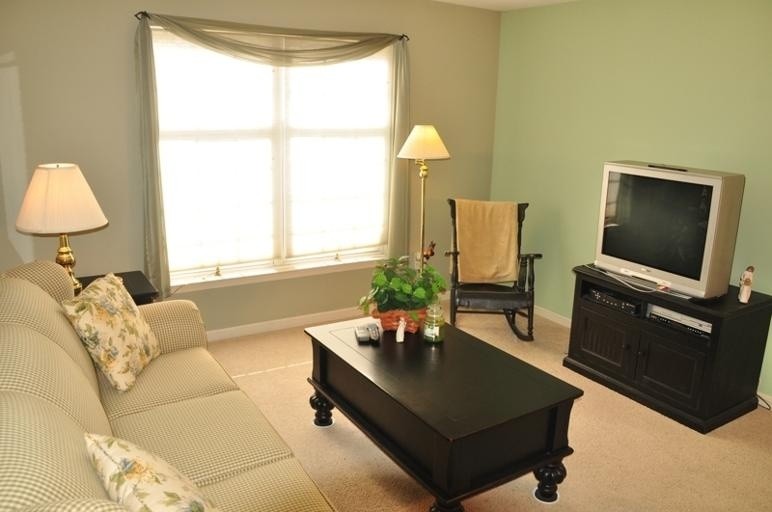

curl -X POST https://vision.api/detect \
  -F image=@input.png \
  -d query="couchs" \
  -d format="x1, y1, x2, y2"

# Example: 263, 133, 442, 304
0, 259, 337, 512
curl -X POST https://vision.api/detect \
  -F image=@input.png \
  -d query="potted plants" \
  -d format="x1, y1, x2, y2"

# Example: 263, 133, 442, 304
356, 256, 448, 334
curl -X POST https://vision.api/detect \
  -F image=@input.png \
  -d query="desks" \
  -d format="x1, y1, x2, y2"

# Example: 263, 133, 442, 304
75, 269, 159, 305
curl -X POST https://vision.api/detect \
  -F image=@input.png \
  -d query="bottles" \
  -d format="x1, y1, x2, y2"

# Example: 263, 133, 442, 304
423, 300, 447, 342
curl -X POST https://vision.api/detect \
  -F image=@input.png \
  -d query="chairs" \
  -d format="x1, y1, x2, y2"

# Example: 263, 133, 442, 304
448, 196, 543, 342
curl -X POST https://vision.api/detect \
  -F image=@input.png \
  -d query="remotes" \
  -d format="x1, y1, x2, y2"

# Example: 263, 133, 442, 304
356, 326, 370, 341
368, 323, 380, 342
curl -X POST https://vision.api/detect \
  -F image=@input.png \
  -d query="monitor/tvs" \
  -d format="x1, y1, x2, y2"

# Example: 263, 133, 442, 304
593, 159, 745, 300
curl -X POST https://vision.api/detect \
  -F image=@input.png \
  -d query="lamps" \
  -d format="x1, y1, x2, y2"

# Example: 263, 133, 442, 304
397, 124, 452, 275
19, 162, 111, 295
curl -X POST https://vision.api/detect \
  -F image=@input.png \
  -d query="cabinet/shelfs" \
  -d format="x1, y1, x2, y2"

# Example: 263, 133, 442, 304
562, 261, 772, 435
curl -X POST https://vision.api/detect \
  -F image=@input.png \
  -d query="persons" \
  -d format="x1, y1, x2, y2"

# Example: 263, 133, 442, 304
739, 266, 754, 304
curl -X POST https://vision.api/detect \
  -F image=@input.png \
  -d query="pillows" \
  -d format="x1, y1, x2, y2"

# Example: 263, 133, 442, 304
82, 429, 223, 512
61, 271, 160, 395
